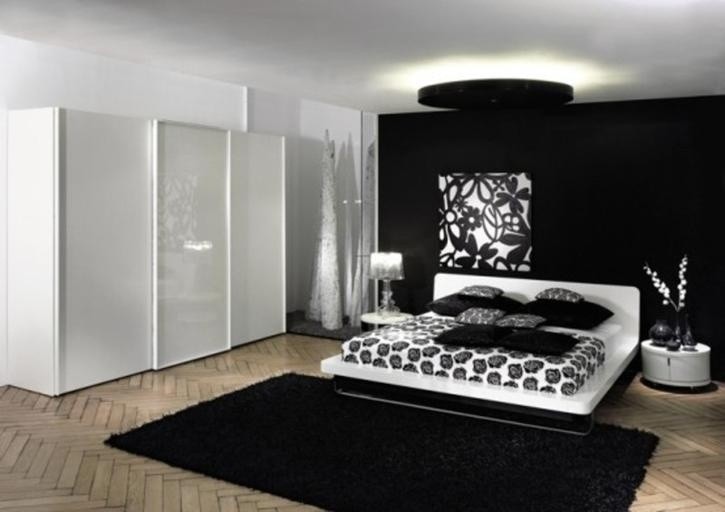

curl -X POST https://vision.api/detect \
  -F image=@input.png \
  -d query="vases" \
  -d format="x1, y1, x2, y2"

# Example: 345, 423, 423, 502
675, 311, 682, 344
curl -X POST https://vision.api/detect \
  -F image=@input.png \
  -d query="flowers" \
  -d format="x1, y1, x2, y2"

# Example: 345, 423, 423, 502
643, 255, 688, 312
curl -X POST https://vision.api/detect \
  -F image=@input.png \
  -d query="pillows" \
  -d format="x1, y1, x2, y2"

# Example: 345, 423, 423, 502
535, 287, 585, 303
505, 298, 614, 330
496, 313, 546, 328
433, 324, 514, 347
426, 293, 524, 317
498, 330, 579, 356
458, 284, 503, 299
455, 307, 505, 326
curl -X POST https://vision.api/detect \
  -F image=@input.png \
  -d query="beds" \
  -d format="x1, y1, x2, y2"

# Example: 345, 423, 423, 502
321, 271, 641, 437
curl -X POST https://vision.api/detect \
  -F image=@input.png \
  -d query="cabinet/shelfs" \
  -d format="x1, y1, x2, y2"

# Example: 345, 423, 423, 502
10, 106, 287, 397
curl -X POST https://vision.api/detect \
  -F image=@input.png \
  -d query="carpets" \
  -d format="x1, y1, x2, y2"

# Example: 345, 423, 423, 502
103, 372, 661, 512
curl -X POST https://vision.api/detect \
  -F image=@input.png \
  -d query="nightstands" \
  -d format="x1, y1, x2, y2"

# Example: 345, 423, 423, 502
641, 339, 711, 389
360, 312, 413, 329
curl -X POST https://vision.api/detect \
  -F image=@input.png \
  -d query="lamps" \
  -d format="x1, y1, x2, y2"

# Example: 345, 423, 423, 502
417, 79, 574, 109
369, 252, 405, 315
